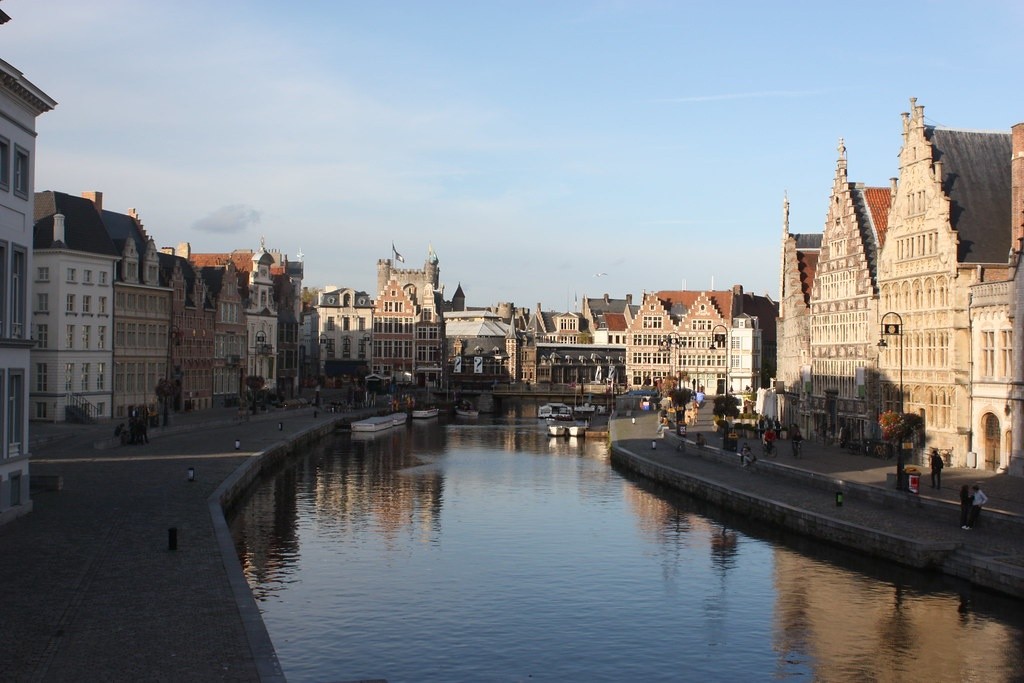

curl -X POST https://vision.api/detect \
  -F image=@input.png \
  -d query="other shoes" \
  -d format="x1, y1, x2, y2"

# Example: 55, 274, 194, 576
961, 525, 972, 530
931, 485, 936, 488
743, 463, 747, 468
936, 487, 940, 490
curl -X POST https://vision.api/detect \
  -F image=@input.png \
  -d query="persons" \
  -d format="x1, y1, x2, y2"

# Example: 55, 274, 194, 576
656, 384, 802, 467
960, 484, 988, 530
931, 450, 943, 490
114, 415, 149, 448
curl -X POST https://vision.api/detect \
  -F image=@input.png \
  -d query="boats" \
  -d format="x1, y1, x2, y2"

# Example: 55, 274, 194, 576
387, 412, 407, 426
455, 408, 480, 416
537, 366, 608, 437
411, 408, 440, 418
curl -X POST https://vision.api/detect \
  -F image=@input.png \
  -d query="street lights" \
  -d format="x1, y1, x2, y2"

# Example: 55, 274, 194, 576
664, 331, 682, 420
315, 333, 330, 401
252, 329, 268, 416
361, 332, 373, 360
656, 335, 671, 395
707, 324, 729, 449
876, 312, 906, 490
161, 324, 182, 426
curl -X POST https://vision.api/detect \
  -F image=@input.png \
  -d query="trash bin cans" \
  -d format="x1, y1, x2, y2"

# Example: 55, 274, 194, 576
678, 421, 687, 437
902, 467, 921, 494
727, 433, 738, 451
148, 414, 159, 428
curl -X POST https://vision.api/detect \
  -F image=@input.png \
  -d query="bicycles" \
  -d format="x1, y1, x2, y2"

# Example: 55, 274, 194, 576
792, 439, 805, 460
760, 440, 779, 460
840, 435, 894, 461
